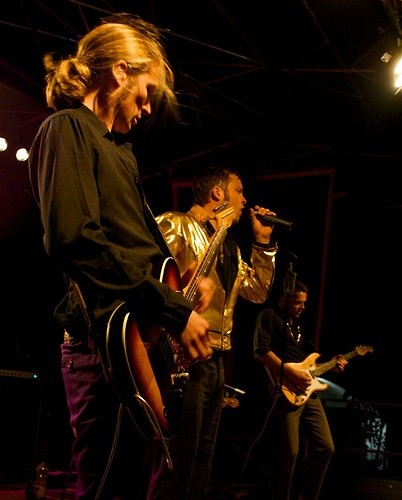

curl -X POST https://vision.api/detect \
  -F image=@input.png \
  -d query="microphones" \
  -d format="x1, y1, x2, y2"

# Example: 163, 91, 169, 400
254, 211, 293, 230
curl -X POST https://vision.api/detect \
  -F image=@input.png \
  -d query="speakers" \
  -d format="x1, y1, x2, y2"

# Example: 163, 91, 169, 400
0, 369, 42, 482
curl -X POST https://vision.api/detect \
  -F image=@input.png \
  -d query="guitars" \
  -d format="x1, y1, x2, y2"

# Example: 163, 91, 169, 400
278, 343, 374, 408
103, 200, 237, 430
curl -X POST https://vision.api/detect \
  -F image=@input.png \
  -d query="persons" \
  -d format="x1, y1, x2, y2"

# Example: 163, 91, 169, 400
28, 22, 215, 500
254, 281, 348, 500
153, 165, 279, 500
